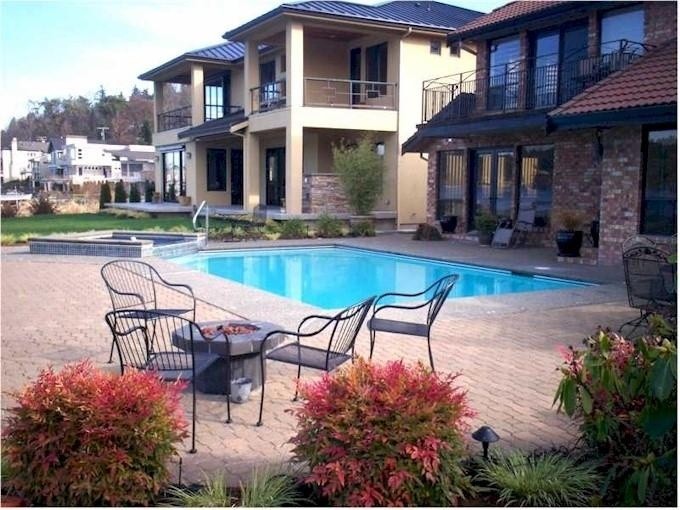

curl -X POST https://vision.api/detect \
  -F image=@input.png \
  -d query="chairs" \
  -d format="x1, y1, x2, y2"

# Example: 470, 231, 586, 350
106, 310, 233, 453
618, 232, 678, 341
257, 295, 377, 425
367, 273, 459, 385
101, 259, 196, 370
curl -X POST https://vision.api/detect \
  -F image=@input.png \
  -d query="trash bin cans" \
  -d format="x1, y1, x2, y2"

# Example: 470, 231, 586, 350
152, 192, 160, 204
230, 376, 252, 403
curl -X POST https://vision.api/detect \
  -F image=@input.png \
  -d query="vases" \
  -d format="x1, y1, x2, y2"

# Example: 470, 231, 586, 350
440, 215, 458, 235
151, 191, 160, 203
590, 220, 600, 248
178, 196, 190, 206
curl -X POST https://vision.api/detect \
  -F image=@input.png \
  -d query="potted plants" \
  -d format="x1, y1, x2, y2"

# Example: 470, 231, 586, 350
474, 207, 496, 245
329, 128, 385, 232
554, 207, 586, 257
